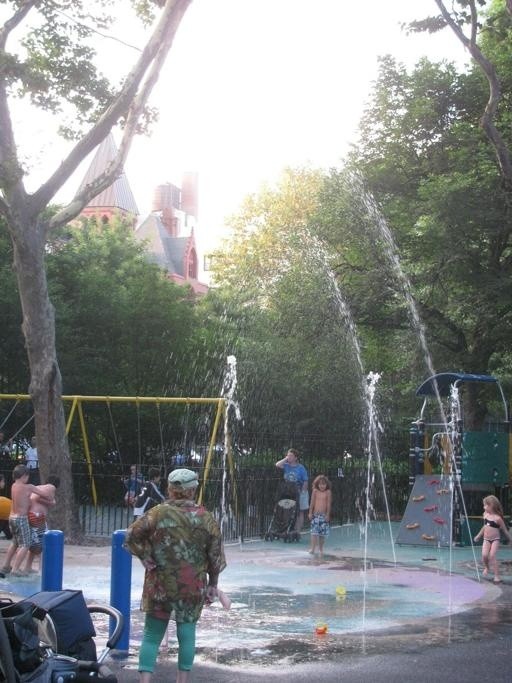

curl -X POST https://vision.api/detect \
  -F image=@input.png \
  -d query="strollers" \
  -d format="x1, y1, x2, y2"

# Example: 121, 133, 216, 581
0, 580, 132, 683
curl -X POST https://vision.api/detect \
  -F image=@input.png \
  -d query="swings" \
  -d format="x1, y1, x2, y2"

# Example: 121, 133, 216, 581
106, 395, 143, 503
0, 396, 35, 451
158, 398, 190, 477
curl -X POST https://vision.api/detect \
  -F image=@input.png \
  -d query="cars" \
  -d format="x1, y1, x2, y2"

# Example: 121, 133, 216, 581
145, 442, 252, 465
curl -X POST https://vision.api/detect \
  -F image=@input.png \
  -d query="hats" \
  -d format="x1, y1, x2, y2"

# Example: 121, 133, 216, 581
167, 468, 200, 490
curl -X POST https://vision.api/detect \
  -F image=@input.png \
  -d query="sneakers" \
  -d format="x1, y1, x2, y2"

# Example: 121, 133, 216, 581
1, 565, 37, 577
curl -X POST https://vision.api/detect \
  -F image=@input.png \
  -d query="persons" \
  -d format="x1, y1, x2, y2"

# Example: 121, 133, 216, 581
133, 468, 166, 523
309, 475, 332, 553
472, 495, 510, 583
1, 474, 15, 540
275, 449, 308, 532
123, 465, 144, 504
122, 469, 227, 682
3, 464, 50, 576
19, 474, 61, 572
